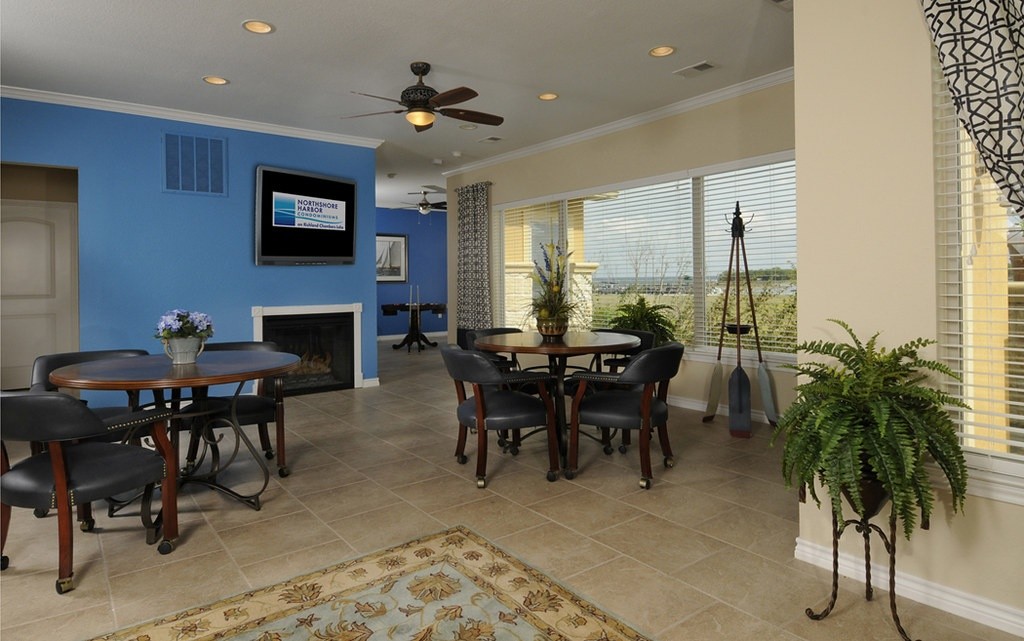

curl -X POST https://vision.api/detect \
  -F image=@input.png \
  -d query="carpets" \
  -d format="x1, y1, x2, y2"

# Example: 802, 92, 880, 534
87, 522, 659, 640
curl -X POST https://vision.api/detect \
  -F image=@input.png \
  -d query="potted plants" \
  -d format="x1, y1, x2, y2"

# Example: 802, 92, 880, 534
769, 317, 970, 543
605, 295, 679, 347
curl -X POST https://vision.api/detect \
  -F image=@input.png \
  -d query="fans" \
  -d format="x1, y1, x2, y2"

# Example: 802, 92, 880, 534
340, 61, 504, 133
390, 190, 447, 214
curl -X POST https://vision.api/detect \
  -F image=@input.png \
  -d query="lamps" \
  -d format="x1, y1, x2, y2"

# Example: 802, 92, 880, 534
406, 107, 436, 126
418, 206, 431, 214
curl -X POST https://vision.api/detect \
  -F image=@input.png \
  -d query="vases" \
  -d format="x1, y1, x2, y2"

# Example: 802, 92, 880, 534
536, 315, 569, 337
162, 335, 205, 364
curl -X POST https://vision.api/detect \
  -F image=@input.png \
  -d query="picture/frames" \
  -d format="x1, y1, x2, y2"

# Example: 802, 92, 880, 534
376, 232, 409, 284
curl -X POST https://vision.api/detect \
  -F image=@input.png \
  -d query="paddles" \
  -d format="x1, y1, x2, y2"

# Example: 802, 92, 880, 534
740, 236, 781, 428
702, 236, 736, 422
727, 236, 753, 439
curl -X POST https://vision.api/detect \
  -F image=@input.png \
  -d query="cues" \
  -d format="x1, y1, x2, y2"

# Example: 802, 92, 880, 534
407, 285, 413, 353
416, 285, 422, 352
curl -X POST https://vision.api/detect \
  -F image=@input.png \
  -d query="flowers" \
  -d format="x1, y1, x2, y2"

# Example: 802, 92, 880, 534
523, 231, 597, 333
154, 308, 214, 342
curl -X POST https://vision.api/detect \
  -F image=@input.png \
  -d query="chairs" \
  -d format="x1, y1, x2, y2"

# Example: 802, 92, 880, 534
564, 340, 685, 490
466, 327, 557, 456
1, 391, 180, 594
440, 342, 561, 488
562, 328, 655, 455
29, 348, 153, 518
178, 340, 290, 478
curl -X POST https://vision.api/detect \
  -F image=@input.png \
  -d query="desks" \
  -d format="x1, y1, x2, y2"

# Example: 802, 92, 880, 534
48, 350, 302, 546
381, 302, 447, 350
474, 330, 641, 469
